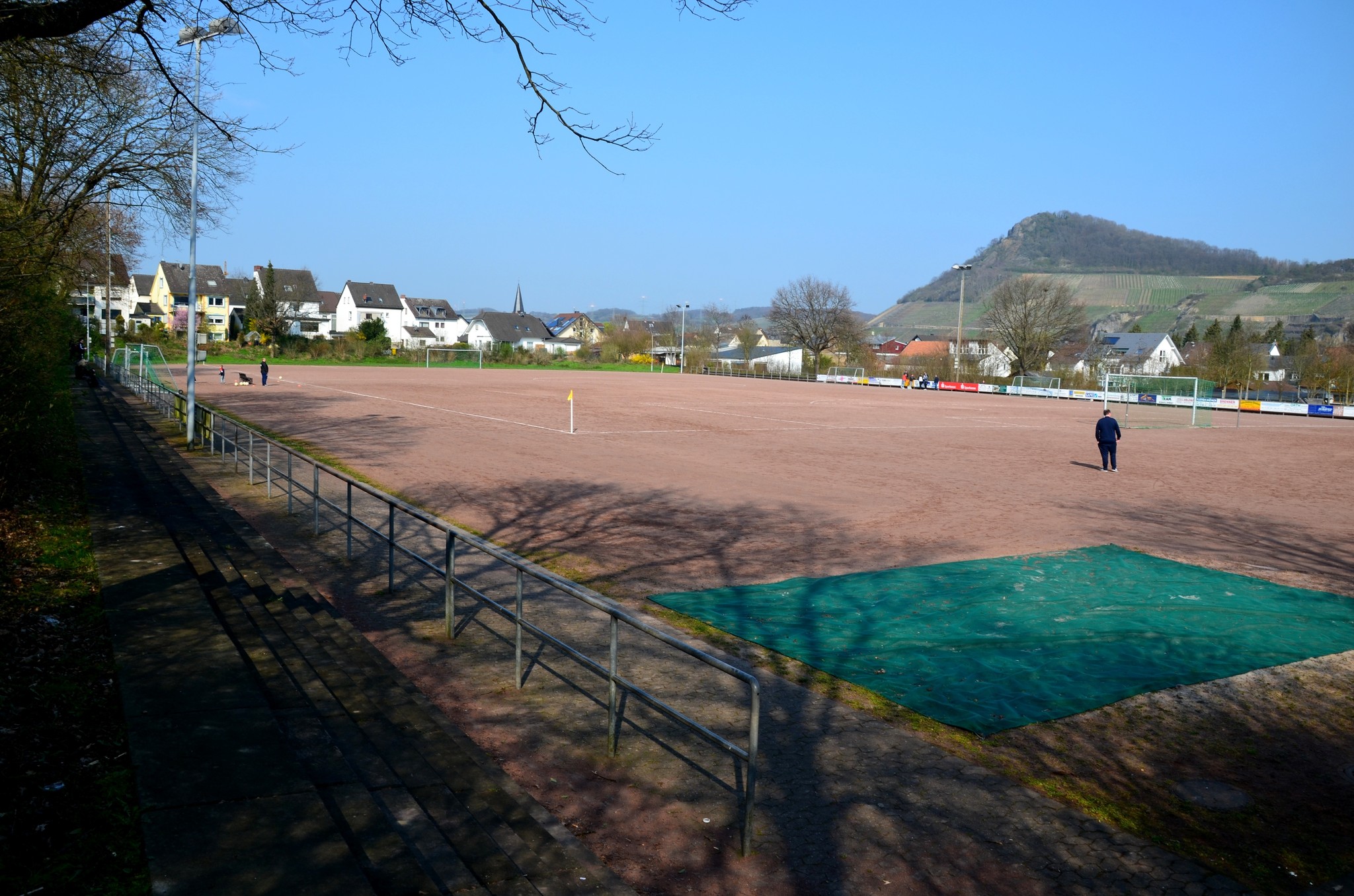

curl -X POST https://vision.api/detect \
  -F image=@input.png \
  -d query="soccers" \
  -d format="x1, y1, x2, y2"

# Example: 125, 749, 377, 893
279, 376, 282, 379
234, 382, 238, 386
243, 382, 246, 385
240, 382, 243, 385
246, 382, 249, 386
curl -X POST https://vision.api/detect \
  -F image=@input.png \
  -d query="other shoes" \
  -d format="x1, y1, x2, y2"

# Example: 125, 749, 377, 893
1100, 468, 1108, 472
1111, 468, 1119, 472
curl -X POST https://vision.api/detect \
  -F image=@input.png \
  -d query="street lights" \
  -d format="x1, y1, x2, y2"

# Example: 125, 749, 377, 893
175, 15, 234, 450
676, 304, 690, 374
952, 264, 972, 383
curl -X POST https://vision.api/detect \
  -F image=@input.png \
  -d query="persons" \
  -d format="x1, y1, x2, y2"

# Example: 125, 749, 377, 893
1095, 409, 1121, 472
924, 372, 929, 377
923, 374, 928, 390
901, 374, 906, 389
934, 375, 939, 390
219, 365, 226, 385
75, 359, 102, 388
260, 358, 268, 386
910, 374, 915, 386
78, 339, 87, 361
703, 366, 707, 372
904, 370, 908, 379
918, 374, 923, 389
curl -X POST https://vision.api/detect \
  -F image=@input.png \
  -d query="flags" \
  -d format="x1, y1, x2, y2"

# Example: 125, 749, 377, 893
568, 393, 572, 401
